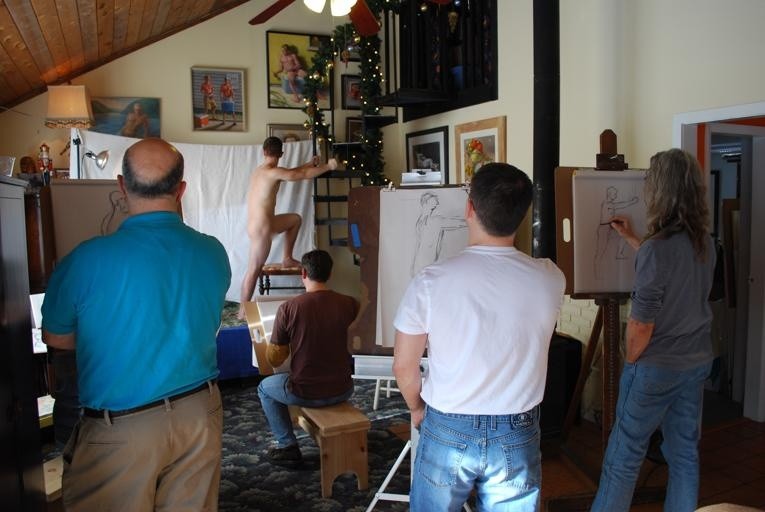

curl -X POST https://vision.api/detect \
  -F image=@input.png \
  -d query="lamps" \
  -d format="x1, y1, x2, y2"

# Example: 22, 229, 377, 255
304, 0, 357, 17
72, 138, 109, 179
44, 80, 96, 129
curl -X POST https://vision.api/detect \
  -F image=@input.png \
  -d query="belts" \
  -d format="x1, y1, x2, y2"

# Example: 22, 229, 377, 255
84, 379, 218, 418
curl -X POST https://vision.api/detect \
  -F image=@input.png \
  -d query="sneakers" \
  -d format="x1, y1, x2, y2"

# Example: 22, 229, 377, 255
266, 447, 302, 469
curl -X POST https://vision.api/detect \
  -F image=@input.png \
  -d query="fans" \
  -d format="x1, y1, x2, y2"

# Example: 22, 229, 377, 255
248, 0, 453, 38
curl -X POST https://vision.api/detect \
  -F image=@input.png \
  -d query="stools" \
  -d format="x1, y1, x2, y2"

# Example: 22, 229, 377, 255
259, 263, 306, 295
44, 454, 65, 503
289, 403, 371, 498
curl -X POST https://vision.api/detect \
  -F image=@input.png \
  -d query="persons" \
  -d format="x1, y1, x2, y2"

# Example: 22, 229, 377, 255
592, 181, 641, 265
273, 43, 329, 104
588, 148, 719, 511
220, 76, 238, 125
41, 137, 232, 511
201, 74, 218, 122
255, 250, 362, 464
116, 103, 151, 137
238, 136, 339, 322
409, 191, 469, 276
99, 188, 132, 239
393, 161, 569, 511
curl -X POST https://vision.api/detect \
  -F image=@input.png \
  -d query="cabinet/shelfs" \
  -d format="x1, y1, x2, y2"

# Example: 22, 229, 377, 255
0, 176, 49, 512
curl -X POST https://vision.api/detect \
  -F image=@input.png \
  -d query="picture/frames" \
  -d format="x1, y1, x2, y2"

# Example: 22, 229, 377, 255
722, 198, 739, 308
710, 169, 721, 237
454, 116, 507, 184
266, 124, 311, 141
339, 49, 364, 168
405, 125, 449, 184
190, 65, 249, 133
265, 31, 334, 111
